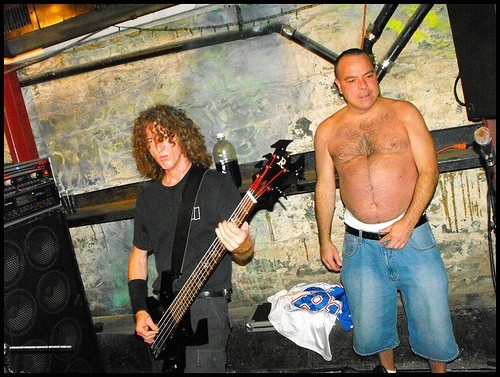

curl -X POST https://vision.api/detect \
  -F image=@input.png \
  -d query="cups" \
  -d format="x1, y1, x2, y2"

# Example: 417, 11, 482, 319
61, 189, 75, 209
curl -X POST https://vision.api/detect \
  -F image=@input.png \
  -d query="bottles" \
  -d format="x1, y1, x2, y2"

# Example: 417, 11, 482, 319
213, 133, 242, 188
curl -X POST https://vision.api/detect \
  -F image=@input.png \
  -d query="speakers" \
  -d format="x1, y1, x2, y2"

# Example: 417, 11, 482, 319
446, 4, 496, 121
3, 204, 106, 373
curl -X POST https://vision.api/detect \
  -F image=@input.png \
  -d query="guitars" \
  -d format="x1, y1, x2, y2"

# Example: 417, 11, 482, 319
133, 138, 305, 377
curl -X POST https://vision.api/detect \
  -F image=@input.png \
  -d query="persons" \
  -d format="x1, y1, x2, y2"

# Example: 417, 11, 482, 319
313, 48, 460, 377
127, 104, 255, 377
